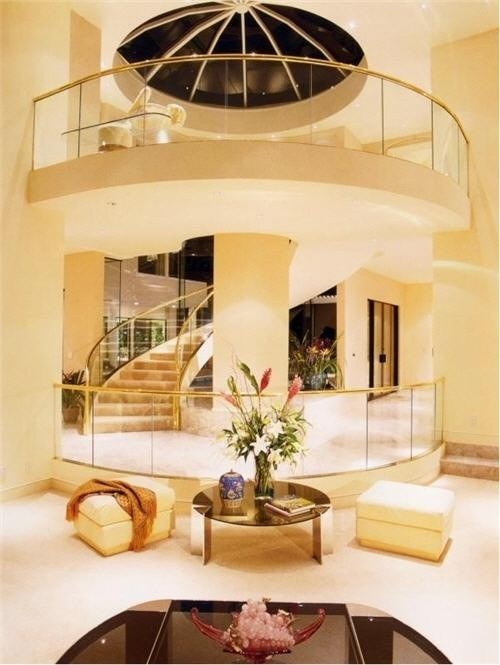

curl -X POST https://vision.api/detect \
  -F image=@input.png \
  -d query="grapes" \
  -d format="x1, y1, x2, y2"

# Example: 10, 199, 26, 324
221, 594, 296, 649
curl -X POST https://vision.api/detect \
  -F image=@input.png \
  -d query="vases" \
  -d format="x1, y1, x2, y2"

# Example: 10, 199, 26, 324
304, 371, 326, 392
63, 406, 79, 425
252, 458, 276, 500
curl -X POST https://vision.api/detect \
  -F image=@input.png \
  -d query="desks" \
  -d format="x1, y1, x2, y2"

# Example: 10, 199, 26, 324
54, 596, 447, 663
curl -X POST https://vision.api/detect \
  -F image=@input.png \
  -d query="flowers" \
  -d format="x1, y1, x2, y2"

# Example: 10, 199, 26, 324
211, 348, 313, 484
288, 331, 343, 386
59, 365, 87, 417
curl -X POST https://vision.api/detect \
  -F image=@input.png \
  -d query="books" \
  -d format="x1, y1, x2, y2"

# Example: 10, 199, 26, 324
263, 494, 319, 518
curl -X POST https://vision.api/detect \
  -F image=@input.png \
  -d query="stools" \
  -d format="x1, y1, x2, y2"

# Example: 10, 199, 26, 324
355, 479, 457, 563
74, 474, 177, 558
